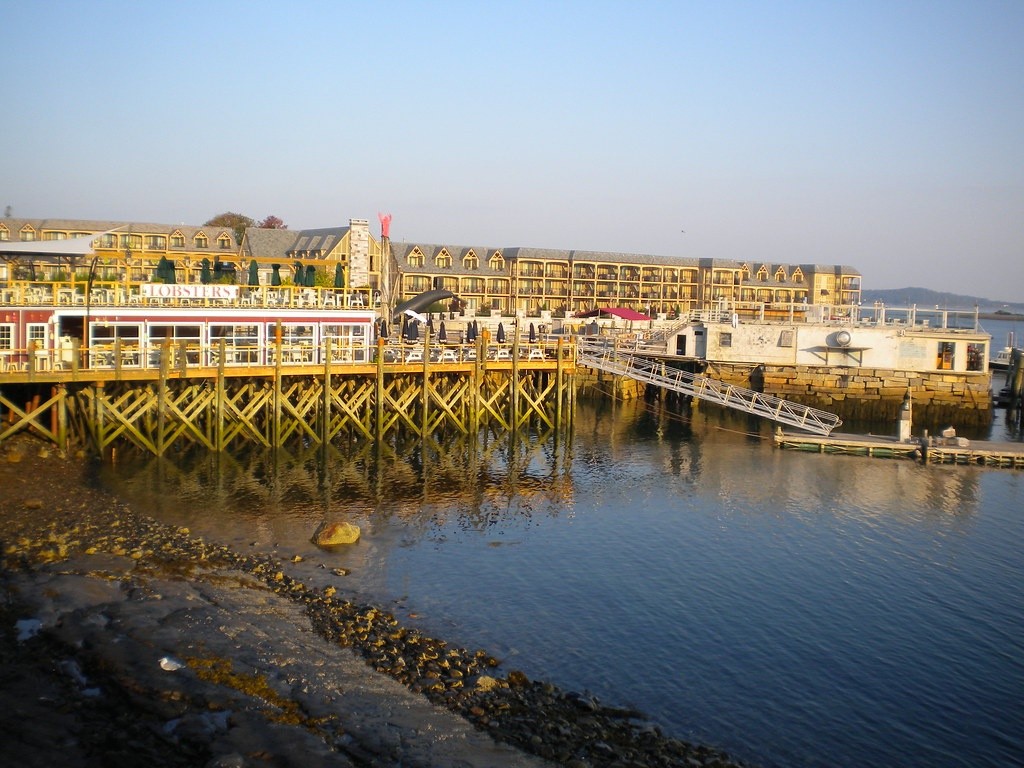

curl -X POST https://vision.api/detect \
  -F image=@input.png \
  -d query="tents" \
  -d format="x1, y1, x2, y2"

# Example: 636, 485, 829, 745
570, 307, 652, 336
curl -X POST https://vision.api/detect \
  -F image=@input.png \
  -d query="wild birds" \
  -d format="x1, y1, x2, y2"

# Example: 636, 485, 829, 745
158, 656, 186, 674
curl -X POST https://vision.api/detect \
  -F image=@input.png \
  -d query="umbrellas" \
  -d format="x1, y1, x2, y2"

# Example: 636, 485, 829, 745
294, 262, 305, 287
427, 319, 434, 338
200, 258, 211, 284
473, 320, 478, 336
381, 320, 387, 346
467, 322, 475, 343
408, 321, 419, 340
249, 260, 259, 293
440, 322, 447, 344
158, 256, 170, 284
496, 322, 505, 343
335, 262, 345, 294
529, 322, 536, 342
271, 264, 282, 286
305, 265, 316, 287
